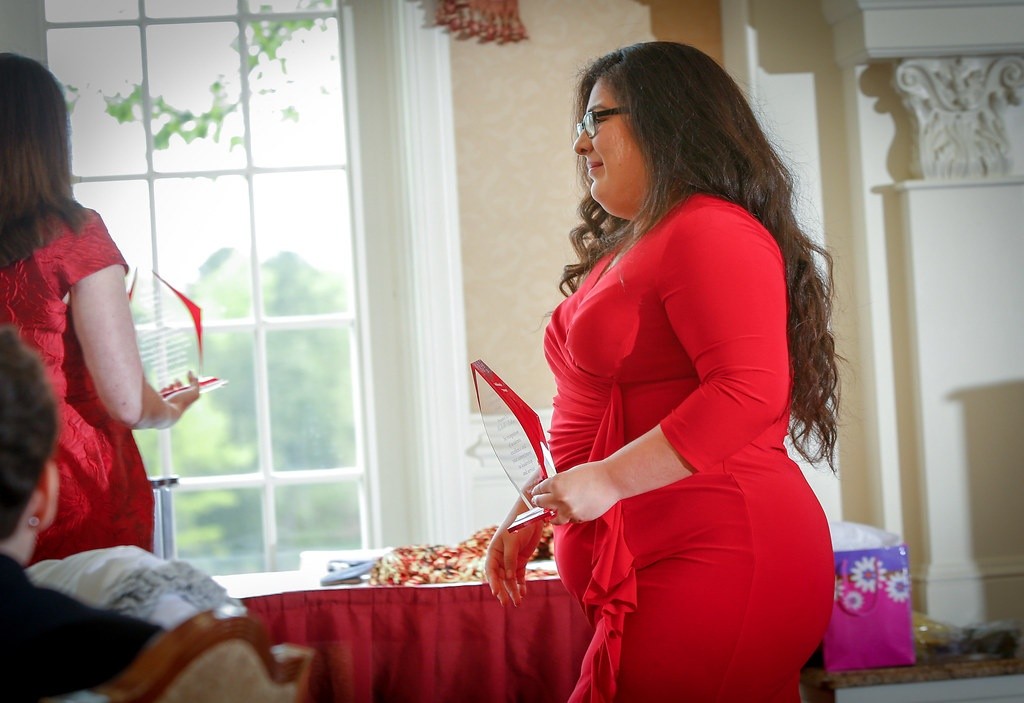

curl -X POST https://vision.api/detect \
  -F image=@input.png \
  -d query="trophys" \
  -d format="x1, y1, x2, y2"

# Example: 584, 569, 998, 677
468, 359, 562, 532
121, 264, 228, 406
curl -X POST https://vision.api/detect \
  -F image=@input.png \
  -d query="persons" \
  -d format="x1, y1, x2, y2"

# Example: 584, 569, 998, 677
483, 40, 841, 703
0, 325, 168, 702
1, 51, 200, 568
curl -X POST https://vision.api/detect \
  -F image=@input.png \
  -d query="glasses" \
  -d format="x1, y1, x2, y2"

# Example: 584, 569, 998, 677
576, 106, 630, 139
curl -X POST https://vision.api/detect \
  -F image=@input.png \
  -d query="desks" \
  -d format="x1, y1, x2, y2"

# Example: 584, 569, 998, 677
241, 576, 596, 703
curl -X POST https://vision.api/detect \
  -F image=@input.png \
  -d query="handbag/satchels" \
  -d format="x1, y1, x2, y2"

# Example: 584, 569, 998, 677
822, 544, 916, 674
